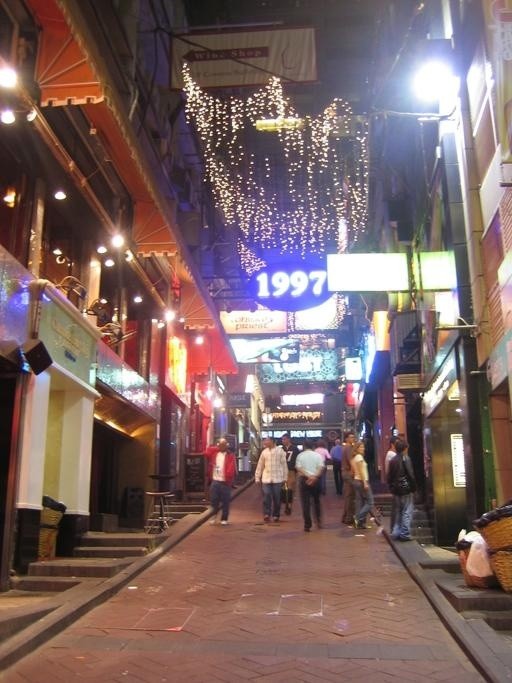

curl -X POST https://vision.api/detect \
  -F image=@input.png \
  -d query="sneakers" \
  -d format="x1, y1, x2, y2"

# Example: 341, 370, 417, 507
221, 521, 227, 525
264, 514, 270, 521
353, 514, 368, 529
272, 516, 279, 521
209, 520, 214, 525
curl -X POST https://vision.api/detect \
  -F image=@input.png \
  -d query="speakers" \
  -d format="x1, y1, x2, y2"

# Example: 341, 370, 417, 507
21, 338, 53, 376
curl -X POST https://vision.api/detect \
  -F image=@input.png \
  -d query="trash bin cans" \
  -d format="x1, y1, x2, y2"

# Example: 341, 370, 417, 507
124, 486, 146, 529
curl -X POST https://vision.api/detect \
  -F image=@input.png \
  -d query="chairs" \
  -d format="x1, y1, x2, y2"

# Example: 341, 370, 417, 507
144, 474, 176, 536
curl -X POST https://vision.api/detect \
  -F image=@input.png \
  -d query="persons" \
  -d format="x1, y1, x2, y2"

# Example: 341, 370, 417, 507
254, 436, 290, 523
340, 431, 356, 525
330, 438, 346, 496
204, 436, 239, 526
384, 434, 401, 534
294, 440, 327, 532
348, 441, 374, 529
282, 433, 301, 499
388, 439, 418, 542
314, 440, 333, 496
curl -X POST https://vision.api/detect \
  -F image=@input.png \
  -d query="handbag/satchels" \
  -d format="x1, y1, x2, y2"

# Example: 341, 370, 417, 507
392, 473, 412, 496
281, 488, 294, 504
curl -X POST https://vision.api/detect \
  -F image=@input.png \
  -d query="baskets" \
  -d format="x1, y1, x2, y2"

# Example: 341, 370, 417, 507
454, 503, 512, 595
